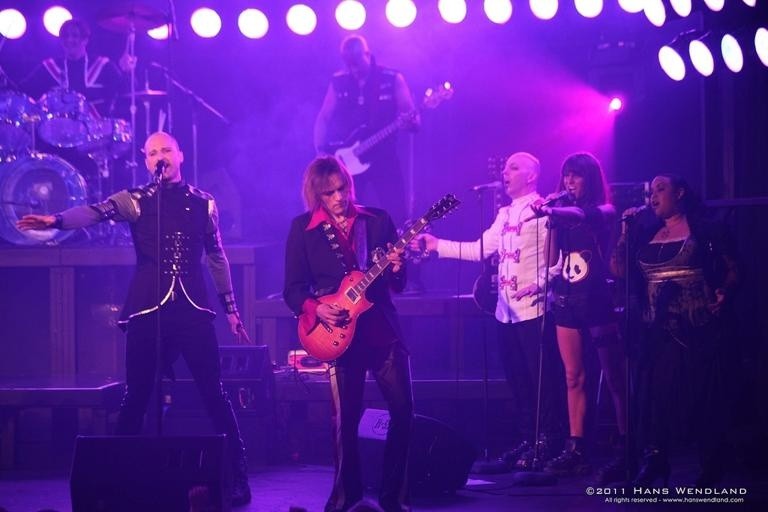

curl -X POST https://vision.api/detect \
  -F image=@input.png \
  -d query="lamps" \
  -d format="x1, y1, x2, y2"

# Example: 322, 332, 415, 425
719, 25, 756, 75
690, 30, 725, 77
659, 30, 698, 83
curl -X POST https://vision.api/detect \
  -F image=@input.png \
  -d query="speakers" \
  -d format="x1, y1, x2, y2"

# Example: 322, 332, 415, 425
219, 345, 277, 466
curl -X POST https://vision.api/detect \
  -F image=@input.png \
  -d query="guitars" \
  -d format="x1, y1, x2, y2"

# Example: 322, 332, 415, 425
327, 79, 457, 177
472, 152, 507, 316
295, 191, 466, 363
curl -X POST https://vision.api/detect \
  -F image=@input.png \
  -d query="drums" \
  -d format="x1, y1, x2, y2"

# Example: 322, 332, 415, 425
39, 87, 96, 147
2, 151, 89, 249
96, 115, 135, 160
2, 88, 38, 147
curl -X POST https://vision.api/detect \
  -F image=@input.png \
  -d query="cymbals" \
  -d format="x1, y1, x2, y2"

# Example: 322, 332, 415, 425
97, 0, 171, 35
121, 89, 172, 100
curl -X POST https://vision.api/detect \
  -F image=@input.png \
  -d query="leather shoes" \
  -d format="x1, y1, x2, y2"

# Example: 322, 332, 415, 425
496, 441, 533, 474
593, 435, 643, 486
544, 436, 602, 477
380, 489, 411, 510
228, 448, 252, 507
323, 482, 363, 512
515, 434, 563, 472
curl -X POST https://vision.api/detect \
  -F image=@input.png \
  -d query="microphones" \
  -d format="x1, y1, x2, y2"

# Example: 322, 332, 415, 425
473, 181, 502, 190
618, 205, 647, 222
542, 190, 568, 206
154, 161, 167, 184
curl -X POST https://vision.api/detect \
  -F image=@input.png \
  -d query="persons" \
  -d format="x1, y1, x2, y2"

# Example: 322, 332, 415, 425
16, 132, 251, 507
530, 153, 636, 477
284, 155, 412, 511
610, 175, 739, 496
312, 36, 421, 288
415, 152, 564, 475
12, 18, 133, 193
